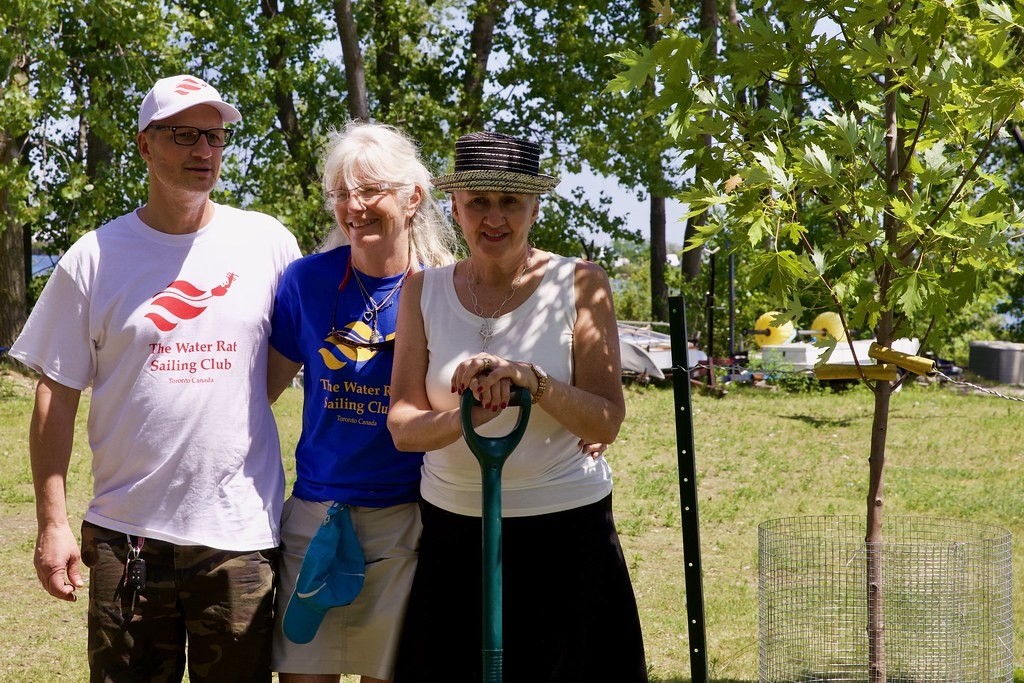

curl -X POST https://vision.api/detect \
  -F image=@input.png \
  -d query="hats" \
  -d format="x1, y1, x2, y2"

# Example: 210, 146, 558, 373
138, 75, 242, 133
280, 500, 365, 644
430, 131, 562, 197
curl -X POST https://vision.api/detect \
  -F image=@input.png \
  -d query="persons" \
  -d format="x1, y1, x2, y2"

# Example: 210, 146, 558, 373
376, 134, 652, 683
228, 122, 484, 682
1, 75, 308, 683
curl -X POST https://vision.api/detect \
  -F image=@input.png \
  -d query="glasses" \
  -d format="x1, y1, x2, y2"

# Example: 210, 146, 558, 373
328, 181, 401, 201
146, 123, 234, 148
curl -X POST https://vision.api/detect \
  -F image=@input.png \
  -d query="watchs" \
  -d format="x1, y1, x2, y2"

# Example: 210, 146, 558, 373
521, 358, 548, 410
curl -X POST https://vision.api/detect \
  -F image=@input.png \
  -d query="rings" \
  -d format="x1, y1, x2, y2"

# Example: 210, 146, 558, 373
482, 356, 491, 369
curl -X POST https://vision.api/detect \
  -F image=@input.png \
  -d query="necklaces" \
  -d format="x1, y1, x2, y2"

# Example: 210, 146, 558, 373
351, 233, 413, 352
348, 242, 413, 322
470, 237, 539, 361
